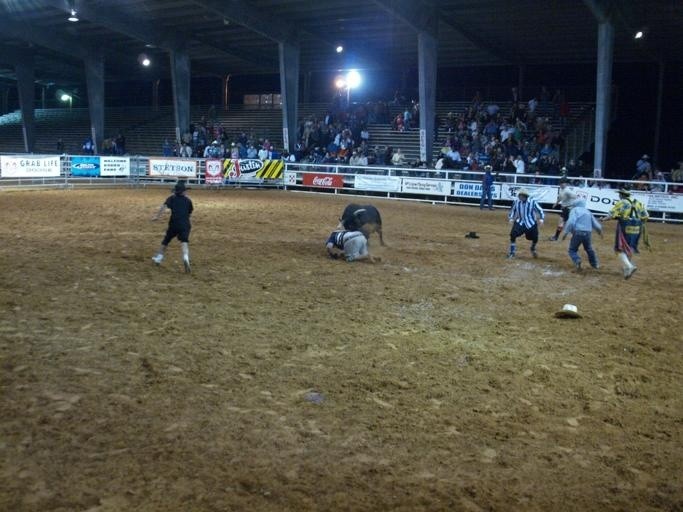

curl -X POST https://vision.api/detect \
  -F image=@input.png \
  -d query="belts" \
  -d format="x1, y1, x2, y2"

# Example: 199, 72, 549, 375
344, 234, 365, 243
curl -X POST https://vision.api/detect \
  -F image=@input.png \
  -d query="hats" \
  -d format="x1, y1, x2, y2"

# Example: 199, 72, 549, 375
484, 165, 493, 170
555, 304, 583, 319
465, 232, 480, 238
172, 182, 191, 194
616, 188, 630, 195
516, 188, 529, 196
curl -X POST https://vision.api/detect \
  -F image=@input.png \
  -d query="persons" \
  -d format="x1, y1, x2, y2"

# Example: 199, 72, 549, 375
150, 183, 194, 273
479, 165, 495, 210
600, 186, 649, 278
508, 189, 545, 258
55, 133, 128, 155
325, 229, 375, 264
161, 86, 429, 169
437, 84, 572, 181
633, 155, 683, 192
550, 177, 603, 269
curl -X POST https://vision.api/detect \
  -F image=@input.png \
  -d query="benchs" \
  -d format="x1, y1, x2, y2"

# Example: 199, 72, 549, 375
1, 100, 601, 178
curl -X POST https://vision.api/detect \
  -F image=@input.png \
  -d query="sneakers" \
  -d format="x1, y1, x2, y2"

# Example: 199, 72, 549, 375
624, 266, 637, 280
152, 257, 160, 265
184, 258, 190, 272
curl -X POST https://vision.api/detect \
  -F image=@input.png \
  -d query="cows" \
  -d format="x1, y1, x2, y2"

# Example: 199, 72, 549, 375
338, 203, 383, 247
